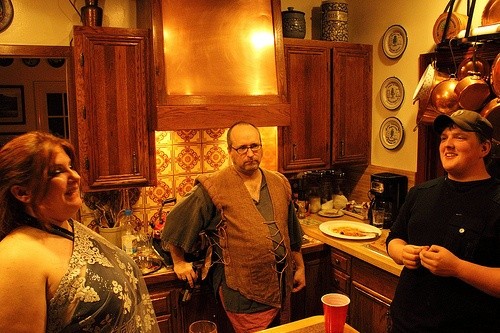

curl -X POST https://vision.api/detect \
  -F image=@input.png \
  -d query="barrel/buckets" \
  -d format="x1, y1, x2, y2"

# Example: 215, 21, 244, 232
320, 0, 349, 42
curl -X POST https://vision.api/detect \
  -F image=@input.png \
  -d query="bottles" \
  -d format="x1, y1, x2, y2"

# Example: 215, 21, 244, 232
120, 209, 137, 257
289, 168, 349, 219
80, 0, 104, 27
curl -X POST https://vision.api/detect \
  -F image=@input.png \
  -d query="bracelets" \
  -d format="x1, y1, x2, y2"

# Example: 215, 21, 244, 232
174, 259, 184, 264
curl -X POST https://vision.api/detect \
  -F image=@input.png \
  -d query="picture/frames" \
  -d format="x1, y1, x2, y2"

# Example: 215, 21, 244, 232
0, 131, 26, 148
0, 85, 26, 125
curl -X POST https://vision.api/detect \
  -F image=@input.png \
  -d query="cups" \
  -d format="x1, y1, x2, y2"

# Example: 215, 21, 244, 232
282, 7, 306, 39
321, 293, 350, 333
188, 320, 218, 333
98, 226, 121, 251
346, 200, 363, 213
309, 196, 321, 213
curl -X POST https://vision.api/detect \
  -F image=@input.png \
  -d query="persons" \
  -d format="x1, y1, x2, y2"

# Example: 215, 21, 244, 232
162, 122, 306, 333
385, 110, 500, 333
0, 132, 160, 333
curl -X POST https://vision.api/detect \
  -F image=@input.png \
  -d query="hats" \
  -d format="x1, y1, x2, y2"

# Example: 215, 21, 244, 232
433, 109, 493, 140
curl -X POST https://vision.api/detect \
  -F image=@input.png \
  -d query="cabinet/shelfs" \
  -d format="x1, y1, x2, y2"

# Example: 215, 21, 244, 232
277, 37, 373, 176
147, 245, 400, 333
69, 26, 158, 192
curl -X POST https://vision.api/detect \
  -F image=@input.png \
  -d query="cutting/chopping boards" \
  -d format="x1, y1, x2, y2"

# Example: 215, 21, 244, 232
369, 235, 389, 256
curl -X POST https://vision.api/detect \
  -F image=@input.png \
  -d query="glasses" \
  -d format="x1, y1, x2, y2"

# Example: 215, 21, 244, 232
231, 144, 262, 154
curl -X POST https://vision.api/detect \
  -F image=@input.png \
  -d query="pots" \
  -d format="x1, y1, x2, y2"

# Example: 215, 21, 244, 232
413, 0, 500, 137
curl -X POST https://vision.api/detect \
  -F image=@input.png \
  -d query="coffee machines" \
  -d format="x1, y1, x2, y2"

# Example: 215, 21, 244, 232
368, 172, 408, 229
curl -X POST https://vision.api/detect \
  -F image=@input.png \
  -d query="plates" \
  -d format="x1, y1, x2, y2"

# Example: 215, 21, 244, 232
135, 257, 163, 275
317, 209, 344, 218
381, 24, 408, 59
379, 117, 404, 150
319, 219, 382, 241
380, 77, 404, 110
